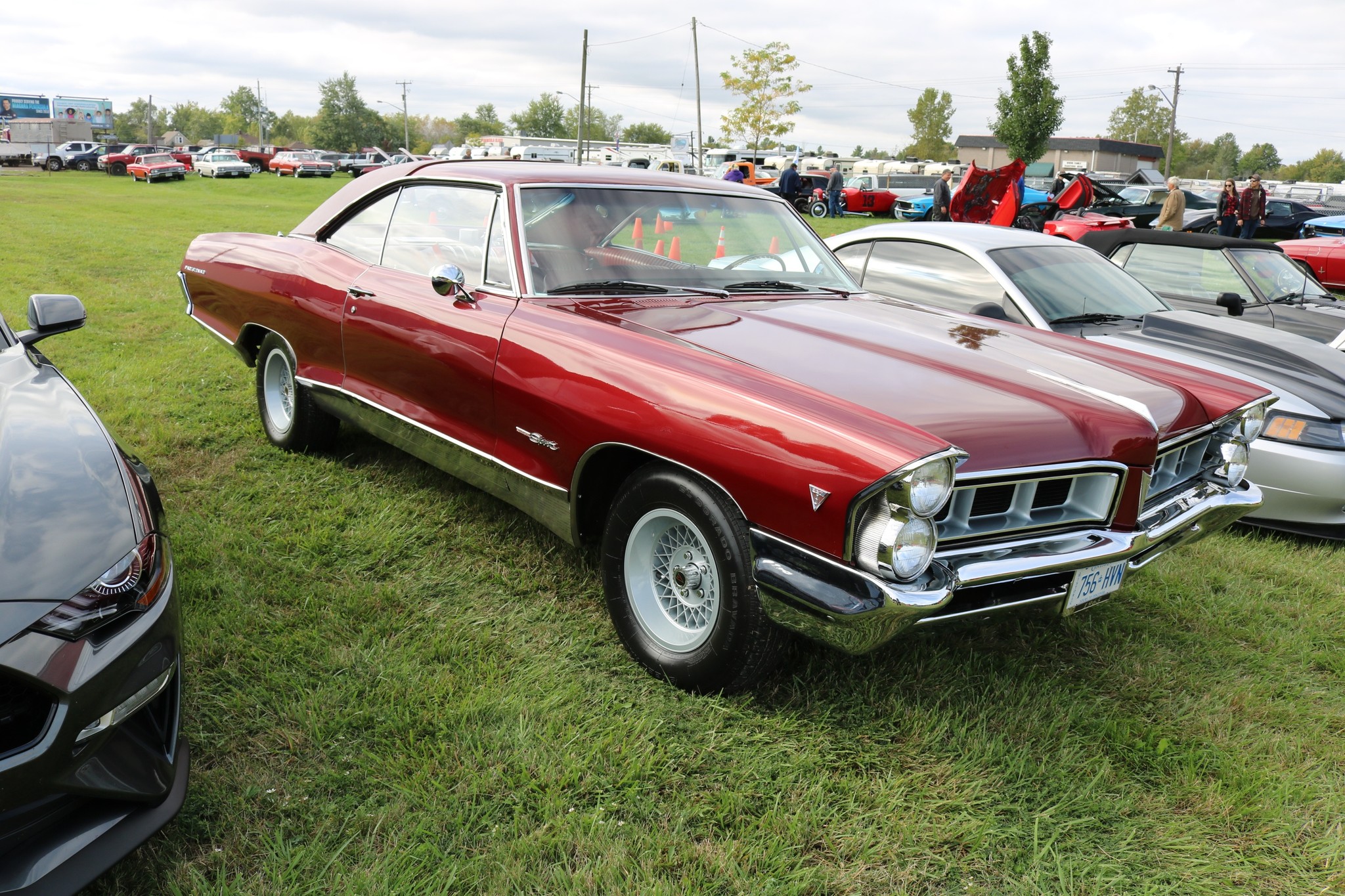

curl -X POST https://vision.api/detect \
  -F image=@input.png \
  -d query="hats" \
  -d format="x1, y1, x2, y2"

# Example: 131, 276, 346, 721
1249, 174, 1261, 180
729, 164, 738, 168
66, 108, 75, 114
828, 165, 835, 171
942, 169, 954, 173
1059, 170, 1066, 175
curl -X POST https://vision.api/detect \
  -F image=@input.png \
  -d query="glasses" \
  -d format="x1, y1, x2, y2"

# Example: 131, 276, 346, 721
1250, 179, 1258, 182
1225, 185, 1231, 187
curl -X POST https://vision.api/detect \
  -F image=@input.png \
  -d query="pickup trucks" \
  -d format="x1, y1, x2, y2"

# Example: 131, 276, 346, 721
97, 145, 192, 176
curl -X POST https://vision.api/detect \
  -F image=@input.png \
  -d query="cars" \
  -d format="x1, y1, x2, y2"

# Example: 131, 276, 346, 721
708, 175, 1345, 542
0, 294, 191, 896
126, 153, 188, 184
194, 154, 252, 179
135, 143, 433, 178
175, 162, 1281, 689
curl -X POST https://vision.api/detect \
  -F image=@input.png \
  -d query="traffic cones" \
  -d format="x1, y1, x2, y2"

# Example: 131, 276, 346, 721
654, 240, 664, 257
634, 239, 643, 251
655, 213, 665, 234
632, 218, 643, 239
664, 221, 673, 231
668, 237, 681, 263
715, 226, 724, 258
768, 236, 779, 255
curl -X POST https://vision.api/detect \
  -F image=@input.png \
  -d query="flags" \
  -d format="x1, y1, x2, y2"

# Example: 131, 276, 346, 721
792, 145, 799, 165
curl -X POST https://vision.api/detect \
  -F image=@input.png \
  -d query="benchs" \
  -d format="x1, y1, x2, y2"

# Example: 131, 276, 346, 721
365, 243, 584, 291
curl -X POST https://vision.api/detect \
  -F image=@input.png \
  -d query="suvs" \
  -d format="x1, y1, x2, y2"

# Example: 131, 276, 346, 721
31, 140, 129, 172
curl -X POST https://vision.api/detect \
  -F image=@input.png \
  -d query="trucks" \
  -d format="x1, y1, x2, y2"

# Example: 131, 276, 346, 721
2, 119, 93, 164
704, 147, 992, 191
428, 145, 694, 174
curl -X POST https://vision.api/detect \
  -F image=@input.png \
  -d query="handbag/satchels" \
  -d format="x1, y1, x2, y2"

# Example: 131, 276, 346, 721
1213, 213, 1219, 222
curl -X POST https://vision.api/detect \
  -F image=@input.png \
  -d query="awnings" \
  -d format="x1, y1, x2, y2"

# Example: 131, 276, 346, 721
1025, 162, 1054, 177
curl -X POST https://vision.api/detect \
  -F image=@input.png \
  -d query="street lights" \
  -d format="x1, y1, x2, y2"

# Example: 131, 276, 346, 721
556, 91, 590, 162
377, 100, 409, 153
235, 114, 263, 146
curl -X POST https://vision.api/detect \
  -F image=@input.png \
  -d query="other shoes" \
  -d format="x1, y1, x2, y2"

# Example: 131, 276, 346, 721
840, 214, 845, 218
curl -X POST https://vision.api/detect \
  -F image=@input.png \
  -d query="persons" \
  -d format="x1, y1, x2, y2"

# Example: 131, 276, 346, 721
0, 99, 17, 119
1050, 171, 1065, 196
57, 102, 113, 124
933, 169, 951, 221
1156, 176, 1186, 231
1237, 174, 1266, 239
1216, 178, 1240, 237
722, 164, 844, 218
